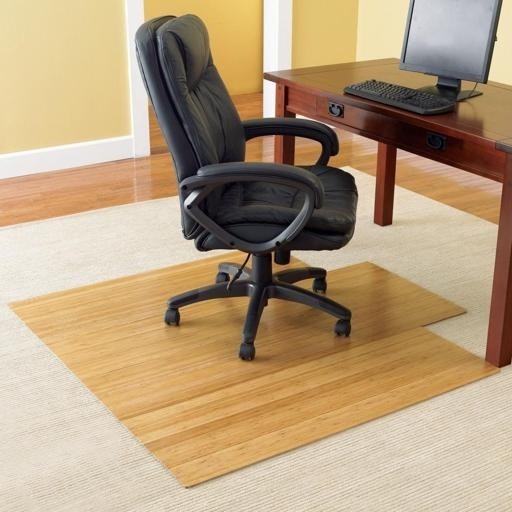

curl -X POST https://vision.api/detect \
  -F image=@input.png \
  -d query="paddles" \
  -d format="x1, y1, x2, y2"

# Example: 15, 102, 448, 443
1, 166, 512, 512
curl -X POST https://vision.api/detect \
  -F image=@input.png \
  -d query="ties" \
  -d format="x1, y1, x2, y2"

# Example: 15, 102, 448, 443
400, 0, 502, 102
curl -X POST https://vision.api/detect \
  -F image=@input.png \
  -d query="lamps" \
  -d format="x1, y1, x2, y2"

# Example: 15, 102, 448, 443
134, 14, 358, 361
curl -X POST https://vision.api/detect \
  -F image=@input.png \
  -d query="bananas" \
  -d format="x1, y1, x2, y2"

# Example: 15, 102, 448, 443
343, 79, 456, 113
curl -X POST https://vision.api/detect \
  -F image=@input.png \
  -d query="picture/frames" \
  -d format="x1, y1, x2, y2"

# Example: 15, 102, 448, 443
263, 58, 511, 368
